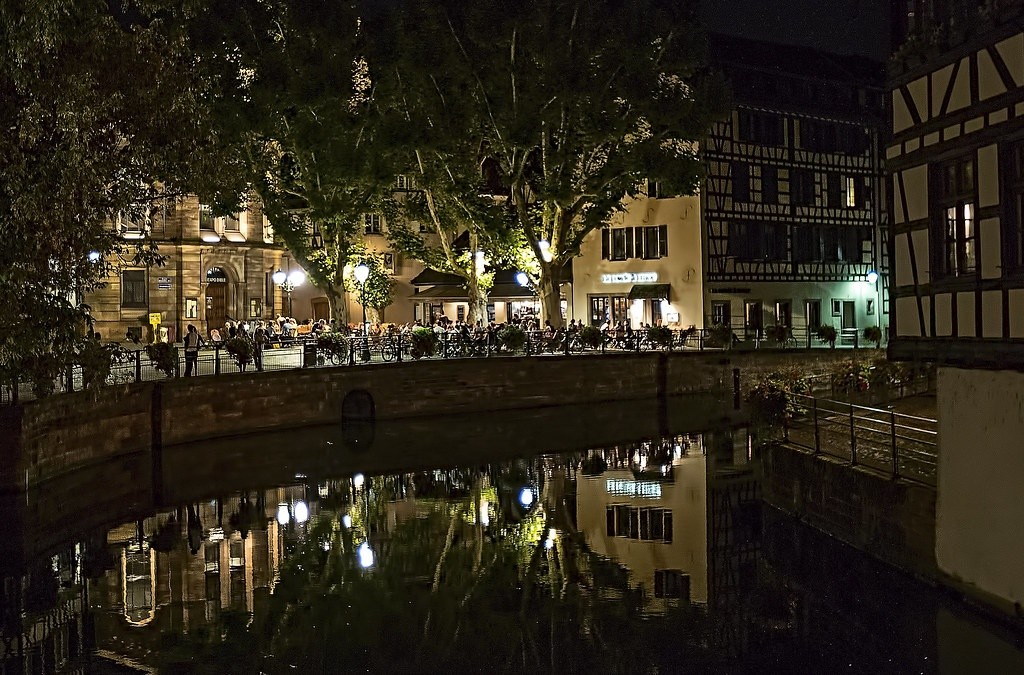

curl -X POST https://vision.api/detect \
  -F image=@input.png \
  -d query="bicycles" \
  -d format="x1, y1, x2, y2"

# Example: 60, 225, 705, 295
621, 334, 648, 351
782, 325, 797, 349
382, 333, 442, 362
314, 336, 346, 365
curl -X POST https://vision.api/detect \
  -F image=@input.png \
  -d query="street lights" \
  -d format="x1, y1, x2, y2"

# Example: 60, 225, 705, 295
356, 261, 372, 360
271, 268, 305, 316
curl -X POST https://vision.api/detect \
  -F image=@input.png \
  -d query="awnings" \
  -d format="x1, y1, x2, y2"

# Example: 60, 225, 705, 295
408, 284, 551, 300
627, 283, 670, 302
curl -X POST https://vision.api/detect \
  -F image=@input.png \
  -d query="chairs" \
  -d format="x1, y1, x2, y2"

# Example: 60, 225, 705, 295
212, 313, 696, 359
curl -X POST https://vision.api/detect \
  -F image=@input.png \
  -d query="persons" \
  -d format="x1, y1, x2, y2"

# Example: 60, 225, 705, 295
79, 433, 705, 579
31, 308, 706, 395
